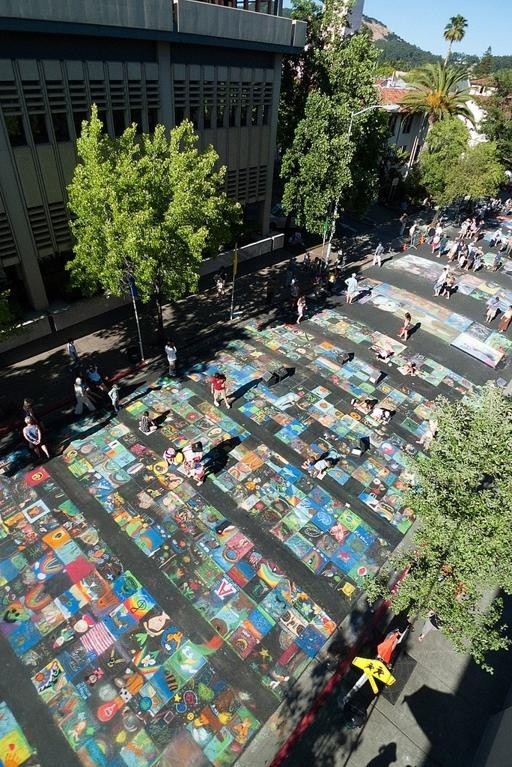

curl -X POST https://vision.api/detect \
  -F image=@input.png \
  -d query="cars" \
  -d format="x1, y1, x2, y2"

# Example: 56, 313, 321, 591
271, 203, 301, 231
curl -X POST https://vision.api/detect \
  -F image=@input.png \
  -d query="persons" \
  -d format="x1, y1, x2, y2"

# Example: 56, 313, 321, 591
165, 341, 178, 376
404, 361, 416, 375
24, 397, 47, 432
141, 410, 157, 432
308, 457, 333, 476
354, 398, 373, 408
163, 441, 206, 486
210, 372, 231, 409
73, 363, 122, 414
373, 409, 390, 424
373, 197, 511, 341
23, 415, 50, 460
214, 247, 358, 303
67, 336, 84, 371
296, 295, 308, 323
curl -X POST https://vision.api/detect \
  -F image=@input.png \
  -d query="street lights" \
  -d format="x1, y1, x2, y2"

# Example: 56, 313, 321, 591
322, 101, 401, 286
